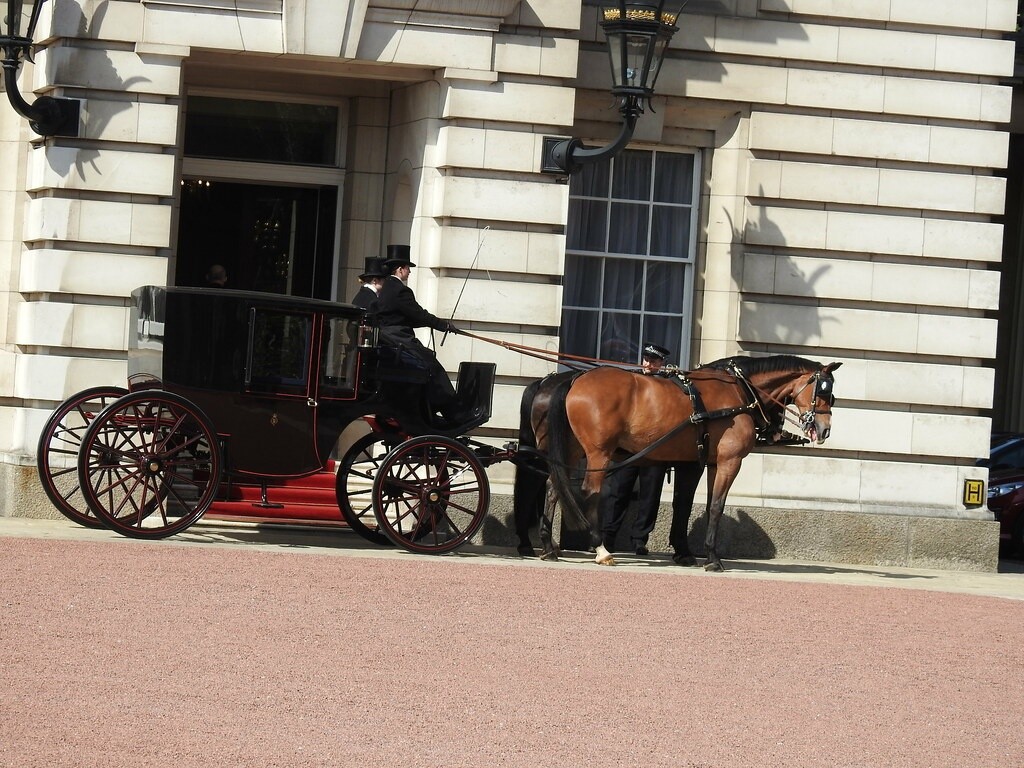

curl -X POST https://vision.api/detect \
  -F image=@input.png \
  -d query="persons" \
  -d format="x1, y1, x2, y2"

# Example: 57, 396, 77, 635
368, 244, 484, 429
205, 264, 227, 288
346, 256, 386, 346
597, 341, 670, 557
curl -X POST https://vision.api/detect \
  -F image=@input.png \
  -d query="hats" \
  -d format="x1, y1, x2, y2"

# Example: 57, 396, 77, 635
644, 342, 671, 360
358, 257, 387, 277
382, 245, 416, 267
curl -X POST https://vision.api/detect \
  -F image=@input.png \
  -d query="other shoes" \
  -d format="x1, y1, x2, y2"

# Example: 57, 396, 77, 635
590, 543, 615, 554
464, 406, 485, 426
635, 541, 649, 556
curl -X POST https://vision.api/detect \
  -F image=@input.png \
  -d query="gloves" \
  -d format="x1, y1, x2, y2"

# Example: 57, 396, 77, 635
447, 321, 455, 333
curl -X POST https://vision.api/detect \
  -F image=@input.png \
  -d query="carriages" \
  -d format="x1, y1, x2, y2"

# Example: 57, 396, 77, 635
35, 285, 845, 569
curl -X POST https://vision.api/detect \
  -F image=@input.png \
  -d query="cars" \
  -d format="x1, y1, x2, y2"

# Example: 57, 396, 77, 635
978, 433, 1024, 552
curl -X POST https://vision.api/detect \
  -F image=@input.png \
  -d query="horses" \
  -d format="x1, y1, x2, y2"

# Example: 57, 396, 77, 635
545, 354, 844, 573
513, 355, 786, 568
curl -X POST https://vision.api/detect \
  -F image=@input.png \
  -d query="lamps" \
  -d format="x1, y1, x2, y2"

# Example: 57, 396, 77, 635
0, 0, 48, 70
597, 0, 688, 116
